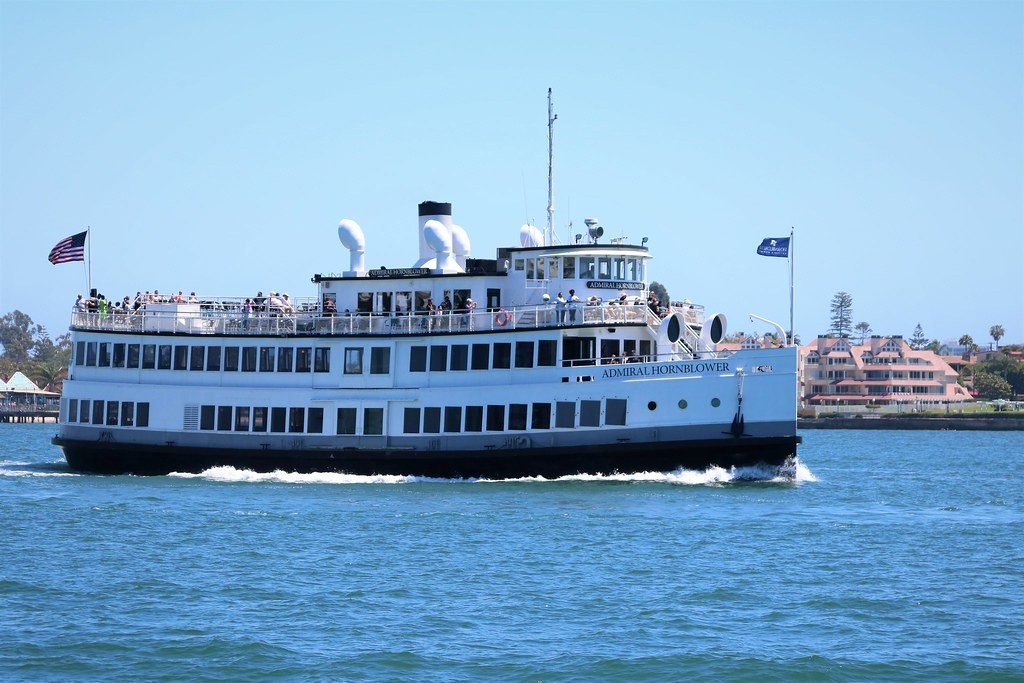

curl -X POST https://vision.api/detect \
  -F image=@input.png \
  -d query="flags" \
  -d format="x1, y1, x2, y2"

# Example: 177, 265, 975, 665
756, 237, 790, 258
47, 230, 87, 266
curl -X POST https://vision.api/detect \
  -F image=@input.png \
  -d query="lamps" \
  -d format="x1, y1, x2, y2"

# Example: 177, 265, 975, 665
641, 237, 648, 246
576, 234, 582, 244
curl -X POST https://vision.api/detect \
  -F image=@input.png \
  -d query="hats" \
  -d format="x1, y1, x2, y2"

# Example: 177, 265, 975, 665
621, 294, 626, 297
593, 296, 597, 300
631, 350, 635, 353
467, 299, 472, 301
270, 292, 276, 295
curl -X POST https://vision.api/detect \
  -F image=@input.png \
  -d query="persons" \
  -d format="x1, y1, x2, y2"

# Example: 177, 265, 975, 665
607, 350, 638, 364
779, 344, 786, 349
555, 289, 698, 327
425, 296, 477, 333
73, 290, 361, 334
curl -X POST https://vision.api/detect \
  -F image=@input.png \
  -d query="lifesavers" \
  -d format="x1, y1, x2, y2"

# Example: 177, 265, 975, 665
496, 309, 510, 326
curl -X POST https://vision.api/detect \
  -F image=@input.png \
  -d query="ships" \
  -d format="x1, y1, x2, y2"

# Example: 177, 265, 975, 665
49, 84, 804, 477
